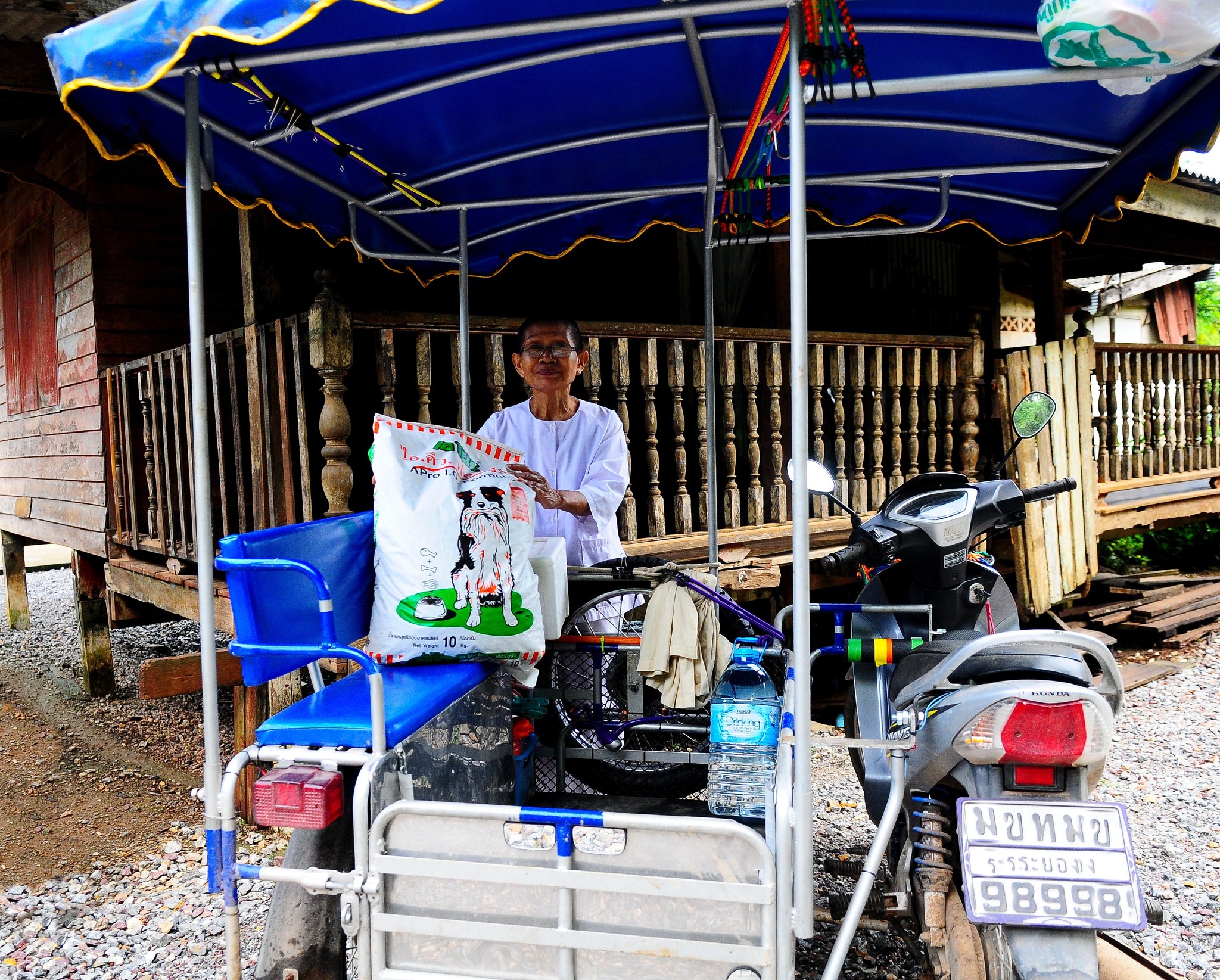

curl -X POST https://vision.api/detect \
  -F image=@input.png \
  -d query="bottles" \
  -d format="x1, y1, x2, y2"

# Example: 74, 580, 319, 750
706, 637, 780, 819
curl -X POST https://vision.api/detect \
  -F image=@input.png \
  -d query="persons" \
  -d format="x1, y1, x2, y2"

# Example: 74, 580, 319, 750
475, 312, 631, 581
42, 0, 1220, 940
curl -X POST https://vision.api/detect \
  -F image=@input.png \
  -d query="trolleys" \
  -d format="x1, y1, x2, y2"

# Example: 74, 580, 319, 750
216, 511, 931, 980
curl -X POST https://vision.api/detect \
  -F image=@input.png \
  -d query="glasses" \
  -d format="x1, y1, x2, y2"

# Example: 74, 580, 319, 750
520, 342, 580, 358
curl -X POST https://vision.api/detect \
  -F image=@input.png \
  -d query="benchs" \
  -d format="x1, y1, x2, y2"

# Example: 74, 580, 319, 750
214, 511, 504, 755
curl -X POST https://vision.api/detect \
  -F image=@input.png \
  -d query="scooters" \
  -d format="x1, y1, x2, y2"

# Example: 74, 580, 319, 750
786, 392, 1162, 980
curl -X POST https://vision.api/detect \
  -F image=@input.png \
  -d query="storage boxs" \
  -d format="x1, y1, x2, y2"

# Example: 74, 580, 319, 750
529, 537, 570, 640
